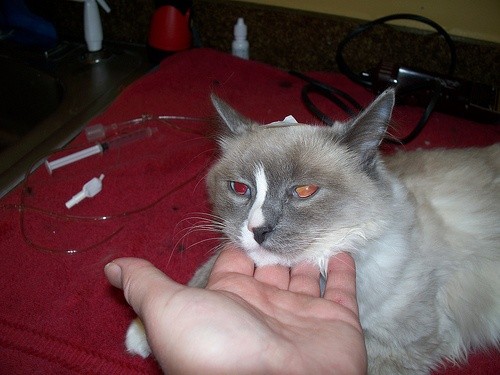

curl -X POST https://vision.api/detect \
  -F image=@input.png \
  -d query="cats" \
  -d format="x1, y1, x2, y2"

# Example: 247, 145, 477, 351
125, 87, 499, 375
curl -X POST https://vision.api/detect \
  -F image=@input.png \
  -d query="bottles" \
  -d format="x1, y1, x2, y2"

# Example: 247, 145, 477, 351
231, 17, 249, 59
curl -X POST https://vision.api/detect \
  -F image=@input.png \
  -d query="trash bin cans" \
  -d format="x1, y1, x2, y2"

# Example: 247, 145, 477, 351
0, 1, 61, 133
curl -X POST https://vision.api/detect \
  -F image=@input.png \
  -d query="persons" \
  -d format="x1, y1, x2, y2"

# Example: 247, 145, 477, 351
103, 235, 369, 375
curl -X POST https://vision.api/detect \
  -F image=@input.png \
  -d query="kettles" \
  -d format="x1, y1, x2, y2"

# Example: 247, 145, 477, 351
147, 0, 202, 57
